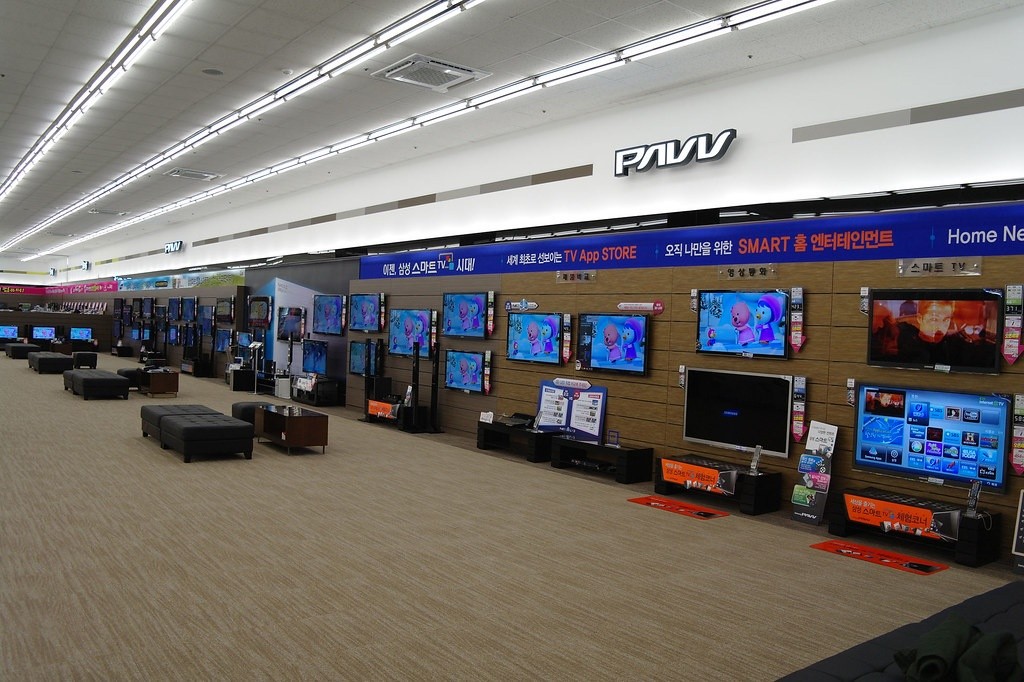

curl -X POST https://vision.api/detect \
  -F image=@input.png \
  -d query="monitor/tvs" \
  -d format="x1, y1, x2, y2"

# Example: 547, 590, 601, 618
695, 290, 791, 360
71, 328, 92, 340
113, 297, 254, 361
32, 326, 56, 340
277, 291, 495, 397
867, 286, 1005, 376
576, 312, 648, 376
0, 325, 19, 339
683, 367, 794, 460
505, 312, 564, 368
852, 381, 1013, 494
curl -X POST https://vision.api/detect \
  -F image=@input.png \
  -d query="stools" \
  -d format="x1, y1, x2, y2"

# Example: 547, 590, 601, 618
138, 404, 226, 444
73, 371, 130, 401
289, 374, 337, 405
158, 412, 255, 464
116, 367, 139, 391
26, 351, 73, 373
61, 367, 109, 397
365, 398, 430, 429
234, 399, 275, 432
4, 342, 40, 362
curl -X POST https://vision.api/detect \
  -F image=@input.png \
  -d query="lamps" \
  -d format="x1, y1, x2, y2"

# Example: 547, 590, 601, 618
0, 0, 834, 261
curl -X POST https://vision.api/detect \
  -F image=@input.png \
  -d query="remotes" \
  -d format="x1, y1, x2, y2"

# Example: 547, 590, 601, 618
533, 411, 543, 428
969, 480, 983, 509
751, 444, 763, 469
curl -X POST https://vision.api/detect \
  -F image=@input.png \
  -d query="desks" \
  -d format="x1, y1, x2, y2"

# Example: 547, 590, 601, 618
471, 410, 555, 462
825, 481, 1006, 568
139, 350, 164, 361
181, 356, 212, 375
73, 350, 100, 369
110, 345, 134, 357
646, 451, 784, 517
49, 339, 74, 353
256, 402, 332, 456
136, 365, 186, 399
545, 433, 658, 489
